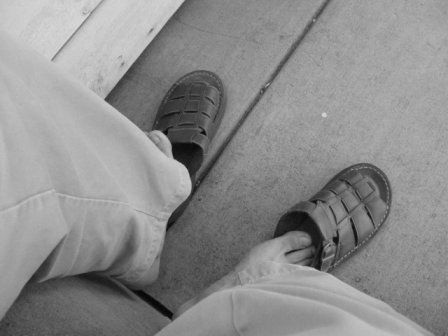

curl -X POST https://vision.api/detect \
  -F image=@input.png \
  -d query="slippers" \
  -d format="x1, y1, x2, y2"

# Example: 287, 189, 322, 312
274, 163, 393, 273
152, 69, 227, 222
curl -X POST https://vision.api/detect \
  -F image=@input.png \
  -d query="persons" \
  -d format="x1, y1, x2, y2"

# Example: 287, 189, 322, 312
0, 16, 442, 336
